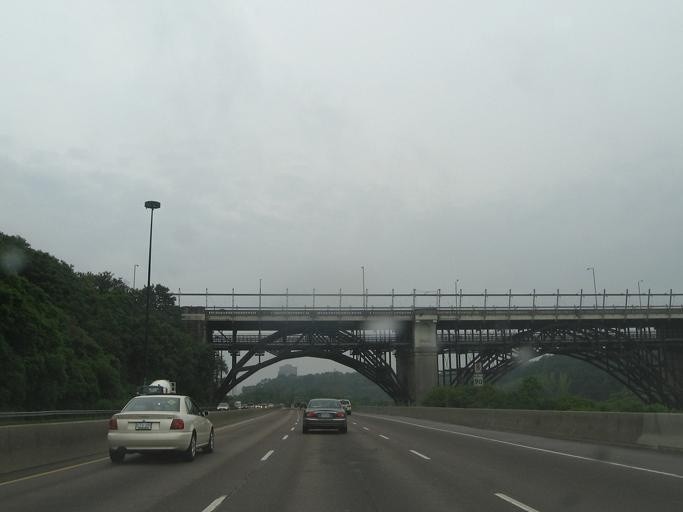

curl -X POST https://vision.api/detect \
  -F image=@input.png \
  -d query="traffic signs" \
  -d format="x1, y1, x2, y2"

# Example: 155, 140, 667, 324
473, 375, 483, 388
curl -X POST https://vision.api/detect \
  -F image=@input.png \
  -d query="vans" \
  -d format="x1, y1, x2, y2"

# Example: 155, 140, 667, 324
338, 399, 351, 414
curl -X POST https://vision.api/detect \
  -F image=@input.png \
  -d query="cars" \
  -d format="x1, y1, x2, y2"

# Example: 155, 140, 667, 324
302, 397, 348, 434
233, 400, 275, 409
216, 402, 229, 411
105, 394, 215, 462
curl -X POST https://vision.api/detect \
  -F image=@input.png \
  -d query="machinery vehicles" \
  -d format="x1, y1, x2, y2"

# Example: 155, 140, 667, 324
136, 379, 176, 396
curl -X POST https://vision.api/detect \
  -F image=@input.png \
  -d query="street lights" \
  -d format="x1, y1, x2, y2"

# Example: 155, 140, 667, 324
133, 265, 139, 290
587, 267, 597, 308
360, 266, 364, 307
141, 199, 160, 385
454, 279, 458, 306
637, 279, 643, 308
